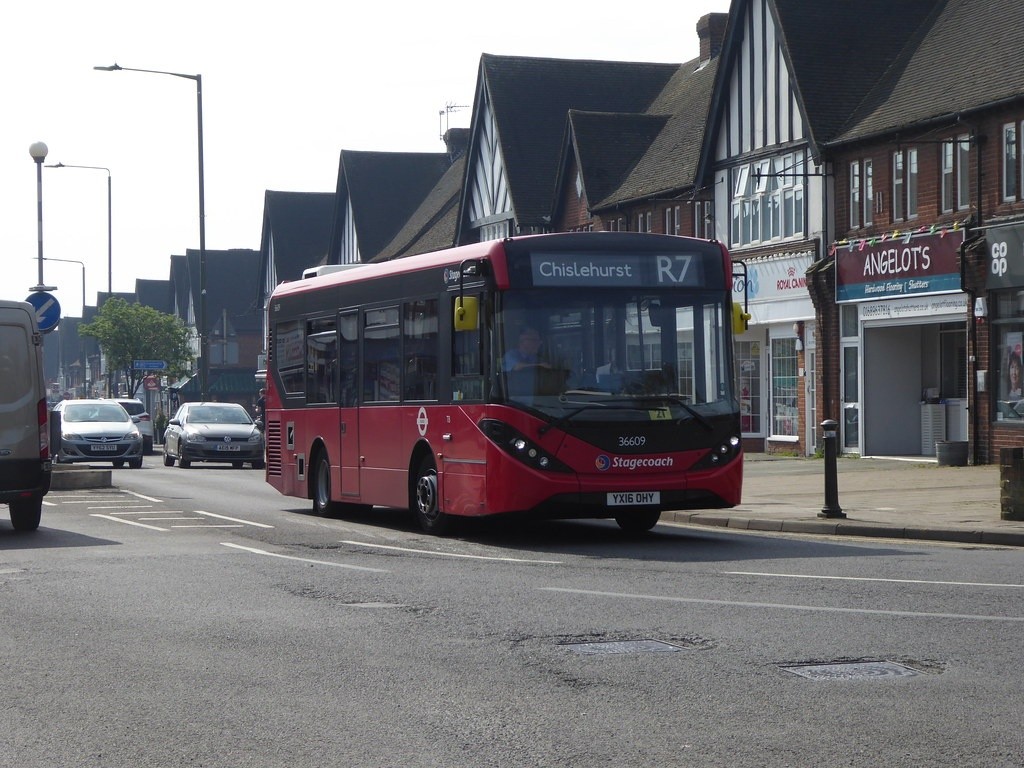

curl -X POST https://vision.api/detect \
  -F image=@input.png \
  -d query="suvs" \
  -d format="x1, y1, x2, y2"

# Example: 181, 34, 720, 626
98, 397, 154, 456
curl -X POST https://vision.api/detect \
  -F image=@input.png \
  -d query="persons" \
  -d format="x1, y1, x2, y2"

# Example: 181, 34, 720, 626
501, 328, 556, 372
1006, 351, 1022, 397
155, 410, 175, 444
255, 389, 266, 449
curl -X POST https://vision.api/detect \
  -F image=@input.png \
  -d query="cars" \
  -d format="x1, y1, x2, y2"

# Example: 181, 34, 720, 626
162, 401, 265, 470
52, 399, 143, 469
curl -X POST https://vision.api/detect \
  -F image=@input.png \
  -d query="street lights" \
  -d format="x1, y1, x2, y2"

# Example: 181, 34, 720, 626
34, 257, 86, 399
94, 67, 210, 402
45, 162, 112, 399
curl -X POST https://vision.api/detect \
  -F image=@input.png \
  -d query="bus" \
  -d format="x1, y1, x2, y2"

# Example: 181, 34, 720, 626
263, 231, 751, 535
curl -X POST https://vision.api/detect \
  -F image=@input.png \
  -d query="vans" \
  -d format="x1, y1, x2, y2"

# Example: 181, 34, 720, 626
0, 301, 52, 531
